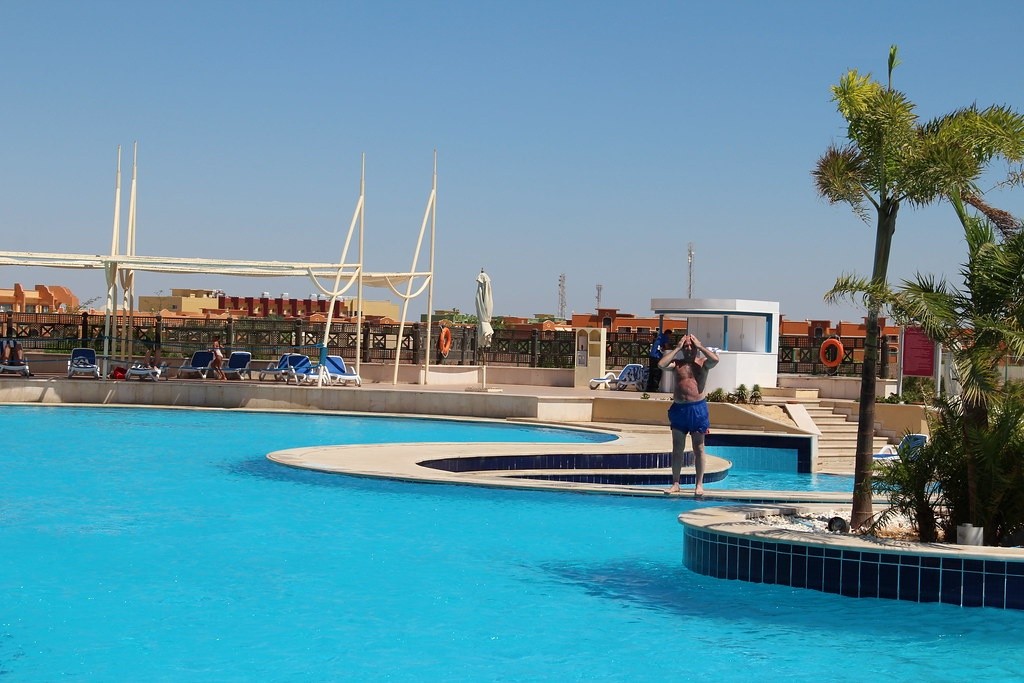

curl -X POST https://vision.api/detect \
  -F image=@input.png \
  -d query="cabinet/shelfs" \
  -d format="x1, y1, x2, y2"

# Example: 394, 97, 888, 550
574, 327, 606, 388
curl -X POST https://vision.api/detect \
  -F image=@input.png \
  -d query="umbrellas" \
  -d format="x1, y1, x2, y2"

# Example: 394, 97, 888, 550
475, 268, 493, 389
946, 337, 963, 417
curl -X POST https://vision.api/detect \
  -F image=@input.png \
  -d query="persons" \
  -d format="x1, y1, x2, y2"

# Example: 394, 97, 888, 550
145, 349, 162, 370
646, 330, 673, 392
3, 343, 35, 376
659, 334, 719, 496
208, 335, 227, 380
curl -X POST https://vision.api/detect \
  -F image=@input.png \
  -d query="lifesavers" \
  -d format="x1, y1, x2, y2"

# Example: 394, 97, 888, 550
439, 327, 451, 354
819, 338, 844, 367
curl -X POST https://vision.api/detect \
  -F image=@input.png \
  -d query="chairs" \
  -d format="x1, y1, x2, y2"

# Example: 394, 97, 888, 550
0, 339, 361, 390
872, 434, 929, 468
589, 364, 652, 390
